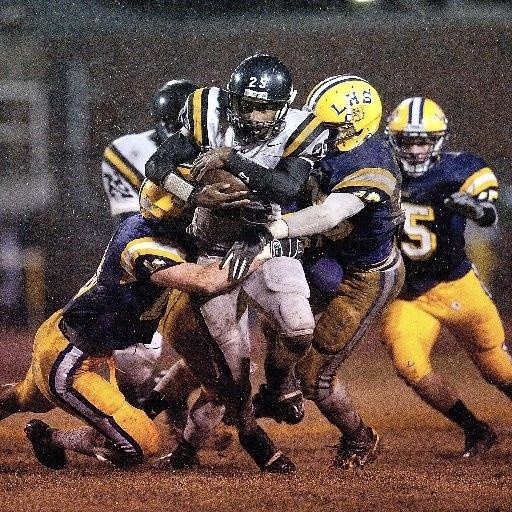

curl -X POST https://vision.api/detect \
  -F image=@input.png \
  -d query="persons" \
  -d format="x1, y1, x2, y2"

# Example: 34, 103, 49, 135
0, 54, 512, 473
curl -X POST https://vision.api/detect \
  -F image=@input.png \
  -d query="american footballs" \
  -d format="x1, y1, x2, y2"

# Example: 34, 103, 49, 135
199, 169, 250, 200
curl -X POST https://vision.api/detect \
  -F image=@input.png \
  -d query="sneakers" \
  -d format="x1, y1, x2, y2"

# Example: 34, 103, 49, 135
170, 450, 198, 467
238, 425, 295, 472
27, 419, 66, 469
445, 399, 497, 457
334, 418, 380, 469
273, 380, 304, 424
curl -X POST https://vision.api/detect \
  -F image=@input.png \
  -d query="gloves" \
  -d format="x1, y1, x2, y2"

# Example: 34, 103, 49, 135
445, 194, 484, 221
219, 224, 273, 281
275, 237, 305, 260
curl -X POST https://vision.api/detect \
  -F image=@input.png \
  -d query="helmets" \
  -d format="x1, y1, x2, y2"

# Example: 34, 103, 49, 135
226, 53, 292, 107
155, 80, 198, 130
305, 75, 382, 153
386, 96, 447, 139
138, 165, 193, 223
